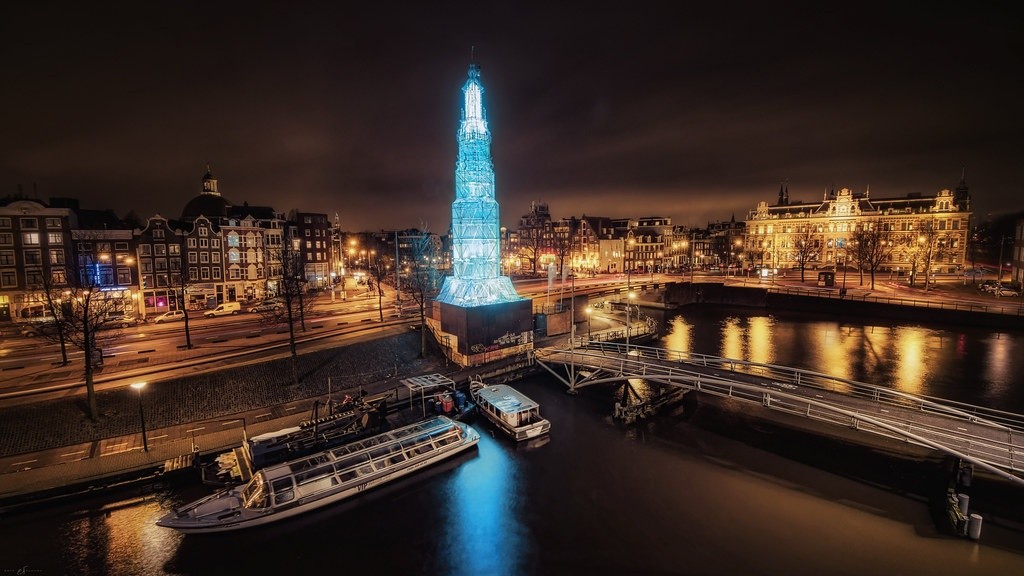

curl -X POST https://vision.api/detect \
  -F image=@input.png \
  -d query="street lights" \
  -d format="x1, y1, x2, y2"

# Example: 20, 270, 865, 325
629, 292, 637, 329
585, 306, 592, 341
129, 379, 148, 452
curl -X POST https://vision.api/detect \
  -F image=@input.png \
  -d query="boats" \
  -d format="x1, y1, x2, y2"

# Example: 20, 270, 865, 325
467, 380, 552, 441
153, 413, 479, 535
200, 374, 479, 488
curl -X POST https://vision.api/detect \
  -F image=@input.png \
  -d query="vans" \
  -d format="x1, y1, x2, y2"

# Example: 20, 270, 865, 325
203, 302, 241, 318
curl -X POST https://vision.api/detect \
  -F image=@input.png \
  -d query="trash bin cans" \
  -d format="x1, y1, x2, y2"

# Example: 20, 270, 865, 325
91, 349, 104, 369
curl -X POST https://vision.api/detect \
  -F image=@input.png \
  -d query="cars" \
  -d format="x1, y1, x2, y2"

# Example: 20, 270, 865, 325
245, 298, 284, 314
154, 309, 186, 325
979, 280, 1018, 298
95, 315, 139, 330
21, 320, 74, 339
